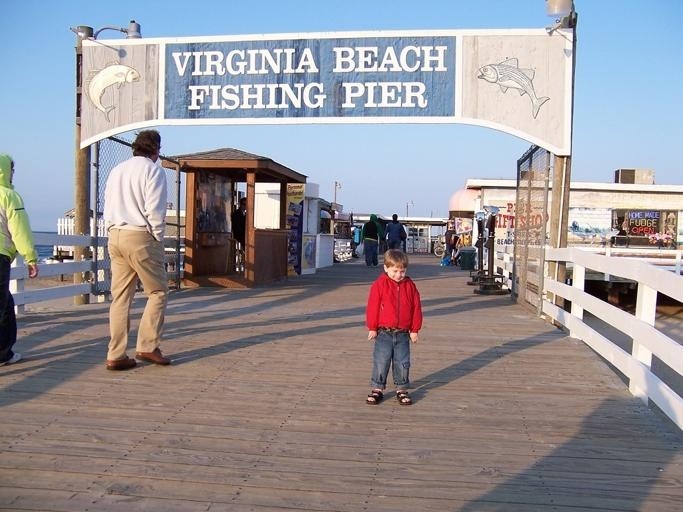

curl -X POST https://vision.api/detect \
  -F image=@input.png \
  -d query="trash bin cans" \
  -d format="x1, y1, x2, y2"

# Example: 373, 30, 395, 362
460, 247, 476, 270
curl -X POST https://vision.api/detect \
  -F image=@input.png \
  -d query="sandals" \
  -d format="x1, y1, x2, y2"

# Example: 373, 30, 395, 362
396, 391, 411, 405
366, 389, 384, 404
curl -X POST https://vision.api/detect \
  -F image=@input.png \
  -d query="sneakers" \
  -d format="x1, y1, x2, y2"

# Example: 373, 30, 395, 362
0, 352, 21, 367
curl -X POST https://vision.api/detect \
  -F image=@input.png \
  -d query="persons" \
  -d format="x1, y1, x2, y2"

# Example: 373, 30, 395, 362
386, 213, 407, 249
0, 154, 39, 367
365, 249, 422, 406
103, 128, 172, 370
231, 196, 247, 250
360, 214, 385, 269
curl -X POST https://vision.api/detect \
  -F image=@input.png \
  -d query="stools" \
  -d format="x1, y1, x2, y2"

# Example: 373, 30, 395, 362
227, 238, 244, 274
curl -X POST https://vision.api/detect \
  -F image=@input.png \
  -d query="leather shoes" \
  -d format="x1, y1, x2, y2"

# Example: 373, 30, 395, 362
106, 355, 136, 370
135, 348, 169, 365
